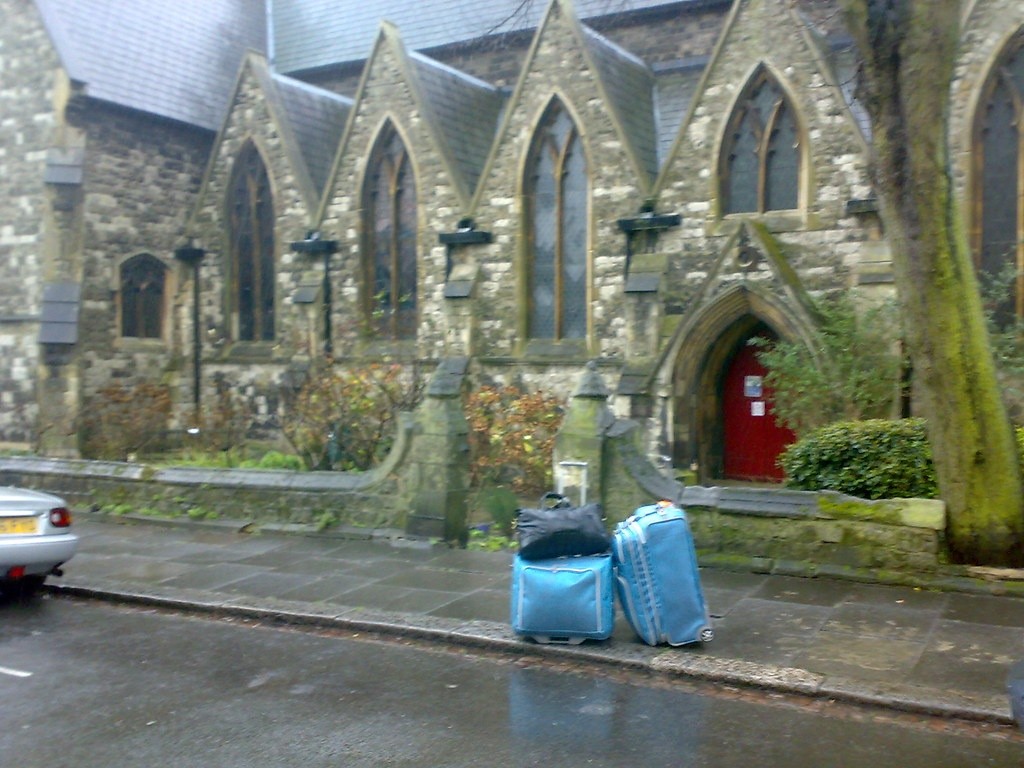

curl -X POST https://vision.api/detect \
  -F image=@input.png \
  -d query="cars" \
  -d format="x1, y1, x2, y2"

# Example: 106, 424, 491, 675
0, 481, 78, 594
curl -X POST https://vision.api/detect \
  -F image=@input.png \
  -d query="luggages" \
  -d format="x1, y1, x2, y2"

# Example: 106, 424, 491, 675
609, 499, 715, 646
512, 461, 615, 644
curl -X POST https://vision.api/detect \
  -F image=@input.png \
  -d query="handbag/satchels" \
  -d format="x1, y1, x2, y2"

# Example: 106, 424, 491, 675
514, 492, 610, 560
